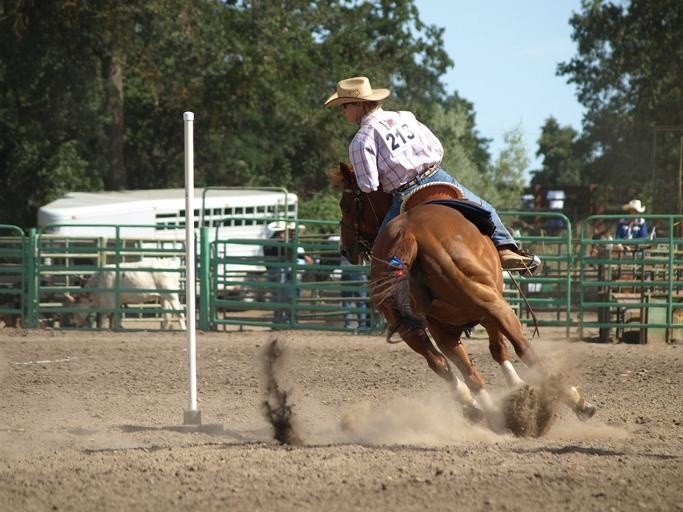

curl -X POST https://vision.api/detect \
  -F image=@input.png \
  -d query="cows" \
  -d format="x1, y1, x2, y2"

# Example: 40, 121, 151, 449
63, 256, 189, 331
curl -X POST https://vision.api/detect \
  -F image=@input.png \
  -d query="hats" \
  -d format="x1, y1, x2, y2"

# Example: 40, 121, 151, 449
268, 221, 305, 237
623, 200, 646, 213
325, 76, 392, 108
297, 247, 305, 253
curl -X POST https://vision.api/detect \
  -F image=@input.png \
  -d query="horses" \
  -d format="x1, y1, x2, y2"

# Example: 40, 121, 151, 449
588, 225, 654, 293
323, 159, 596, 435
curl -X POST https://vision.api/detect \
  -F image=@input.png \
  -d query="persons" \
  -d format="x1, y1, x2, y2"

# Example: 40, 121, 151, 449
615, 199, 648, 257
328, 235, 368, 329
589, 221, 614, 255
323, 76, 533, 271
265, 221, 313, 324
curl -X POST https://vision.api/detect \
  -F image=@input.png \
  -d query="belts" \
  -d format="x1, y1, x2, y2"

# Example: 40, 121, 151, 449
398, 166, 436, 193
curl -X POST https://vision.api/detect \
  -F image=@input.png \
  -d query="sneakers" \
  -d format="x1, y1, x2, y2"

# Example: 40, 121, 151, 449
499, 246, 541, 277
